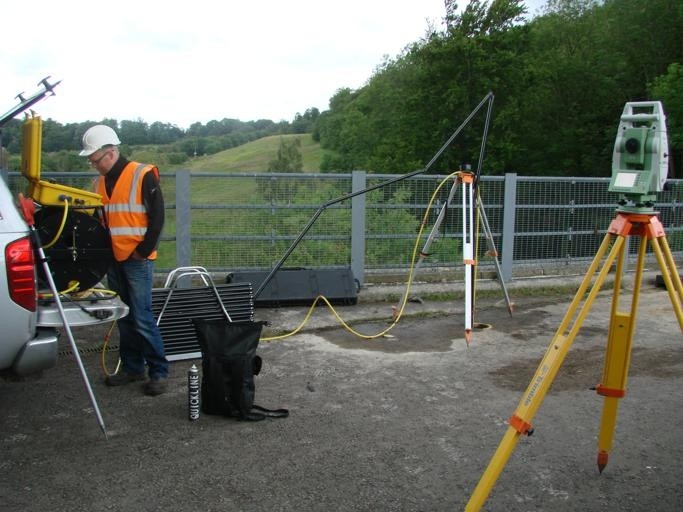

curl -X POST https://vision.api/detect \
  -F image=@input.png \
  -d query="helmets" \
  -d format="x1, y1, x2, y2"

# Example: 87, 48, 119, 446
79, 125, 122, 157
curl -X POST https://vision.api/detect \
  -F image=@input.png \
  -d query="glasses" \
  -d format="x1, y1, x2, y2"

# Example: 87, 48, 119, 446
86, 152, 113, 166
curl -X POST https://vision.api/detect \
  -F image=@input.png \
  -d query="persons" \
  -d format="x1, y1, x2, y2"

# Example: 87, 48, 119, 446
77, 124, 170, 395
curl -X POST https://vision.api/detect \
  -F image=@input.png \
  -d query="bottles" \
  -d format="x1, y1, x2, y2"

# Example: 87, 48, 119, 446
186, 363, 200, 422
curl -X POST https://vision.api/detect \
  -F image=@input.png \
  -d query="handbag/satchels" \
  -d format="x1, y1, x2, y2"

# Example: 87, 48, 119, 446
192, 319, 289, 420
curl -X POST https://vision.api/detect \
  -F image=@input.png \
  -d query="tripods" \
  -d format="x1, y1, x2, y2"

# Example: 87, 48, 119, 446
392, 174, 513, 347
464, 214, 683, 512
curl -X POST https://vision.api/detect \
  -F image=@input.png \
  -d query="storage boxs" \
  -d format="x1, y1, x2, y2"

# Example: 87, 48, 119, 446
20, 117, 102, 217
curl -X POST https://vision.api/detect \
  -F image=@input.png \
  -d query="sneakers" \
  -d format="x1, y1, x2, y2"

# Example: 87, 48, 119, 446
145, 375, 169, 394
106, 368, 146, 386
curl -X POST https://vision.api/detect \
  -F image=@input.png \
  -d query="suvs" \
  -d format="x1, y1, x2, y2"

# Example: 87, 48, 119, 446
0, 77, 130, 389
